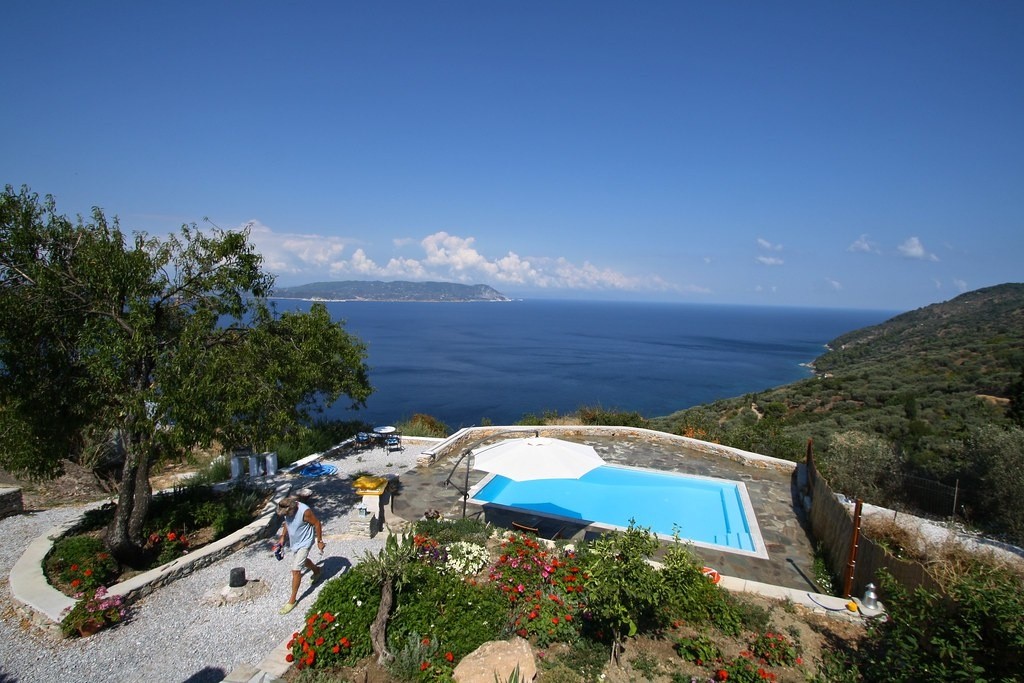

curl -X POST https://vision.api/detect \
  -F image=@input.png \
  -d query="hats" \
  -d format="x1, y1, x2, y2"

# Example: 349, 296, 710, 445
276, 498, 296, 515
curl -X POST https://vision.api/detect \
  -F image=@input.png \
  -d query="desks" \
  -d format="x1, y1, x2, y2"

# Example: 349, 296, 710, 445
373, 426, 396, 448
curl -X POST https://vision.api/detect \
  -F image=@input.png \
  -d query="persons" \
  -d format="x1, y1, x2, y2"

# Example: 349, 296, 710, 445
274, 497, 326, 615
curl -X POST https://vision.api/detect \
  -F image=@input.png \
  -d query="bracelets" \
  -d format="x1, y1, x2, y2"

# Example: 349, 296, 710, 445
317, 541, 323, 543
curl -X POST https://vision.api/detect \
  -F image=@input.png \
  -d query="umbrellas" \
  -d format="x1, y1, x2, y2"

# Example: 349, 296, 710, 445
443, 429, 608, 520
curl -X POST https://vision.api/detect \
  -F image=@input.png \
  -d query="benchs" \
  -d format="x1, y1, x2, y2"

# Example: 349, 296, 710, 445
356, 480, 388, 519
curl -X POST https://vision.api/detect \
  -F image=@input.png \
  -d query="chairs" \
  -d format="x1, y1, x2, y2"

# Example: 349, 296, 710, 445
355, 431, 372, 453
511, 519, 567, 540
383, 431, 402, 456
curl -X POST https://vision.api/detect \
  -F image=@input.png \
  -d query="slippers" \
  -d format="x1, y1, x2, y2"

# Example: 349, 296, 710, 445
278, 599, 298, 615
309, 567, 325, 585
